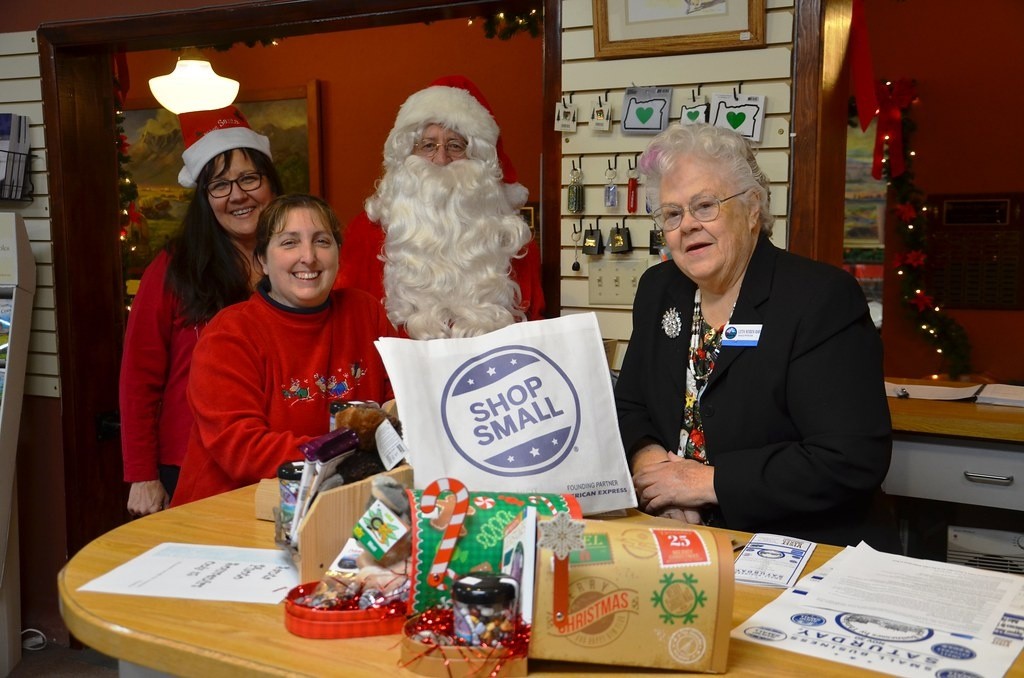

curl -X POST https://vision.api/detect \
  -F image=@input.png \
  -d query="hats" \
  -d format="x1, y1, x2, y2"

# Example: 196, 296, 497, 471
178, 103, 274, 188
384, 74, 529, 209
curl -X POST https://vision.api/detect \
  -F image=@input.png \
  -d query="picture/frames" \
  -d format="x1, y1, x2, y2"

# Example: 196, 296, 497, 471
593, 0, 767, 60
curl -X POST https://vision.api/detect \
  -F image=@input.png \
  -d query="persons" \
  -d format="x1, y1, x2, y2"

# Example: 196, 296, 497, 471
333, 75, 546, 340
120, 106, 284, 517
614, 124, 892, 557
169, 193, 401, 509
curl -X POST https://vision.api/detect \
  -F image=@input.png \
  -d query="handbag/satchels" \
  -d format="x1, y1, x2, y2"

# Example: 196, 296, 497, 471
374, 312, 638, 516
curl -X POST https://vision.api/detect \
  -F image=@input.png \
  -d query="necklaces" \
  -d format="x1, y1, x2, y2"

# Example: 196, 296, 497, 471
689, 286, 741, 380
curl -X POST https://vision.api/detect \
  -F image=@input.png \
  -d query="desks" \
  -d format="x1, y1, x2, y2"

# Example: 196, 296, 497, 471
880, 378, 1024, 563
59, 401, 1024, 678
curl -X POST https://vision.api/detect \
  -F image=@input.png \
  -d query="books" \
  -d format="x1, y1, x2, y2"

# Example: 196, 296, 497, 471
0, 113, 30, 199
884, 381, 1024, 407
0, 299, 12, 407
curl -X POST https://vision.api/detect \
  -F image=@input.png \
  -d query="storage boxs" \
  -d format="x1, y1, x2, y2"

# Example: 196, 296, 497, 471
285, 489, 735, 678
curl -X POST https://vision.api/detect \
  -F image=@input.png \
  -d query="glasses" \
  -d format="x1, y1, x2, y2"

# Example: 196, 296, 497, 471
413, 138, 469, 158
202, 171, 265, 198
649, 188, 748, 231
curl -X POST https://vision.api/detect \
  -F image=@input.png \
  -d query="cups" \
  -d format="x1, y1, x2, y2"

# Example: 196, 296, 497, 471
277, 460, 306, 529
450, 572, 519, 646
